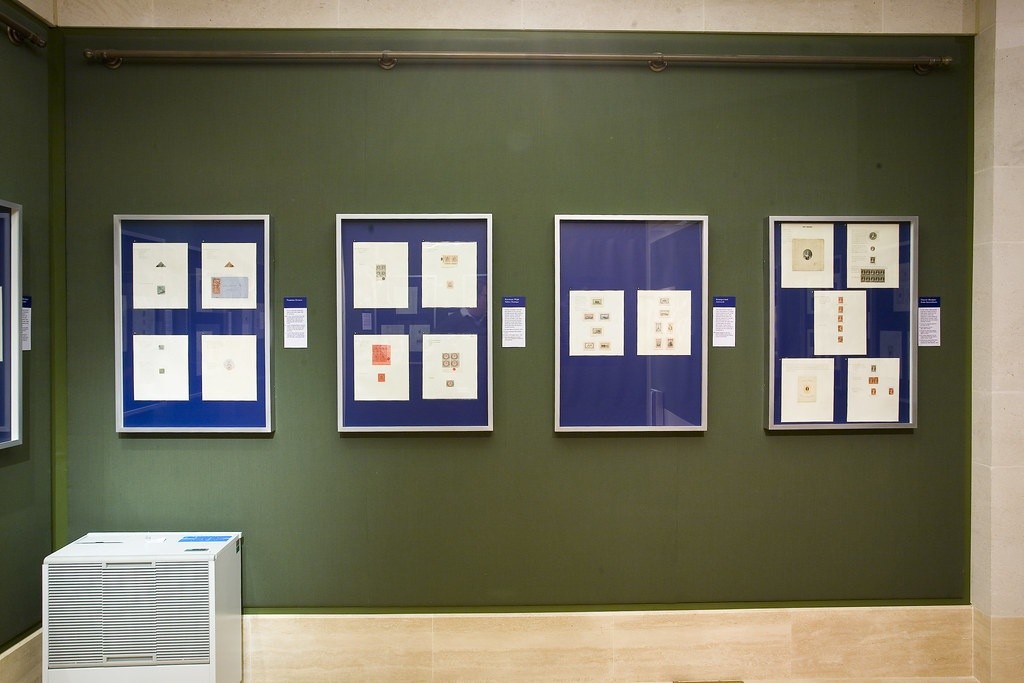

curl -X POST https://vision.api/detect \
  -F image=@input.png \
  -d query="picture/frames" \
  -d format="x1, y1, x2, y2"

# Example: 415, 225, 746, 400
553, 214, 708, 432
0, 199, 24, 450
113, 215, 275, 433
768, 214, 919, 431
336, 213, 493, 432
